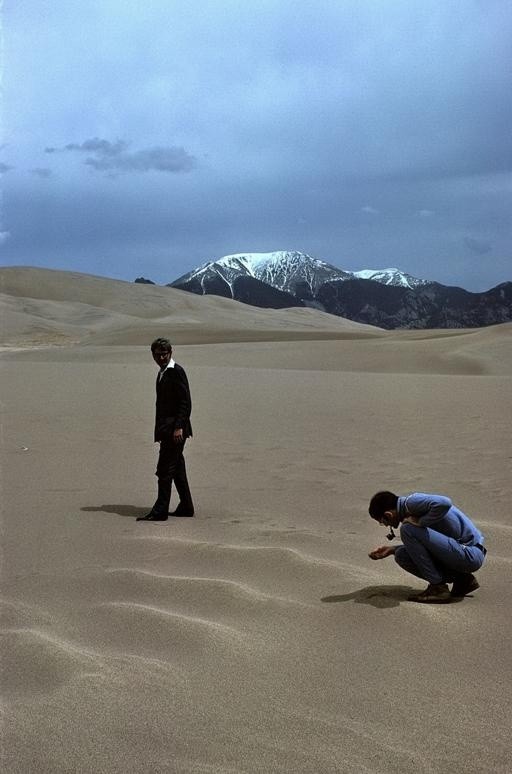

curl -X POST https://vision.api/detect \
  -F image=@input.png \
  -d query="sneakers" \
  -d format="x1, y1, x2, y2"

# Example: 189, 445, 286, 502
450, 575, 479, 596
168, 511, 176, 516
407, 584, 450, 601
136, 514, 154, 521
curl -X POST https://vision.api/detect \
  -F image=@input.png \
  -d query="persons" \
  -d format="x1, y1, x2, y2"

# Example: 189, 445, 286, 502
136, 338, 194, 521
368, 491, 487, 603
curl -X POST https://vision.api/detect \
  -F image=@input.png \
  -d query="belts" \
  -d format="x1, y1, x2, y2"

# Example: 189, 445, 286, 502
475, 543, 487, 554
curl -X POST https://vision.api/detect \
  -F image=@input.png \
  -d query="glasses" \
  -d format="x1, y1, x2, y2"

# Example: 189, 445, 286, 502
161, 352, 169, 359
